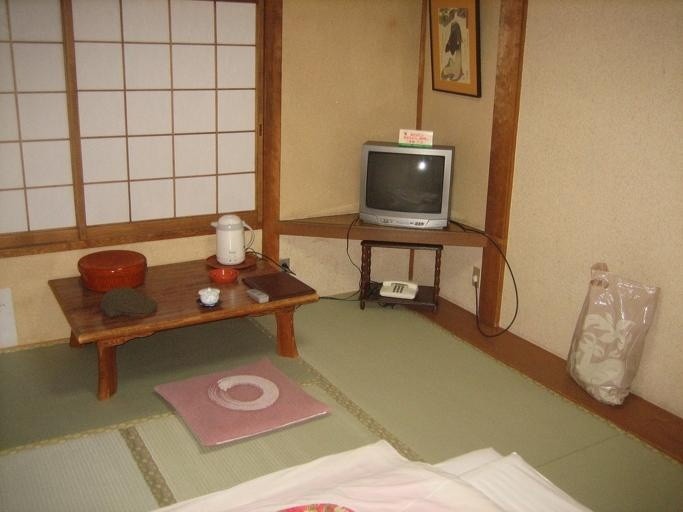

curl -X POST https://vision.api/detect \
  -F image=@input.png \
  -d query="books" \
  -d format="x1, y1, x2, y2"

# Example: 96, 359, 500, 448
241, 271, 314, 299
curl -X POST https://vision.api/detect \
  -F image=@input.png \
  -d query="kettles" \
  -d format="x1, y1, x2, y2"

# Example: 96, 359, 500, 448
209, 213, 256, 265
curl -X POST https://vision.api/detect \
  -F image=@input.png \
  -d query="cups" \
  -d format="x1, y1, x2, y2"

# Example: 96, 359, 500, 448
198, 287, 220, 306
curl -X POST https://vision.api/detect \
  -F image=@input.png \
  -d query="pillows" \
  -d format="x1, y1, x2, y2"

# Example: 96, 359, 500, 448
153, 354, 333, 448
455, 451, 595, 512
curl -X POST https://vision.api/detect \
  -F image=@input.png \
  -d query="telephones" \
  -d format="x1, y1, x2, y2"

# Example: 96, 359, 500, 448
380, 280, 419, 300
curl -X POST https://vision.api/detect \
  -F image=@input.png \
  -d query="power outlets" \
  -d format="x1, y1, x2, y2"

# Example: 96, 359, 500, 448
471, 266, 480, 288
279, 258, 291, 274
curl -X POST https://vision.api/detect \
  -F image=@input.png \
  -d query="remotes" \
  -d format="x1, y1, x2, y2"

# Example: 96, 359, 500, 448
246, 288, 269, 304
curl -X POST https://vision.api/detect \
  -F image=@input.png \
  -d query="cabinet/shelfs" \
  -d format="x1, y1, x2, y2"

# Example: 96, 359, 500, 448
359, 240, 444, 314
262, 222, 510, 331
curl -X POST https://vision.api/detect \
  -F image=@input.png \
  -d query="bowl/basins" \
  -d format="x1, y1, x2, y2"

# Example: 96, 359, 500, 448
207, 268, 240, 284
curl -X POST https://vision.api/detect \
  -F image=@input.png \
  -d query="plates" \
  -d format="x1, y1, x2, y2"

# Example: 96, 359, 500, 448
205, 255, 259, 269
196, 298, 223, 309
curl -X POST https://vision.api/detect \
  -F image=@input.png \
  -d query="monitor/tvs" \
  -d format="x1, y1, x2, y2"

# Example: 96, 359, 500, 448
359, 140, 455, 229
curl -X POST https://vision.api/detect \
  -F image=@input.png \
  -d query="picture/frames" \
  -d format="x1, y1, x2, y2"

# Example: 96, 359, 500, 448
429, 0, 482, 98
